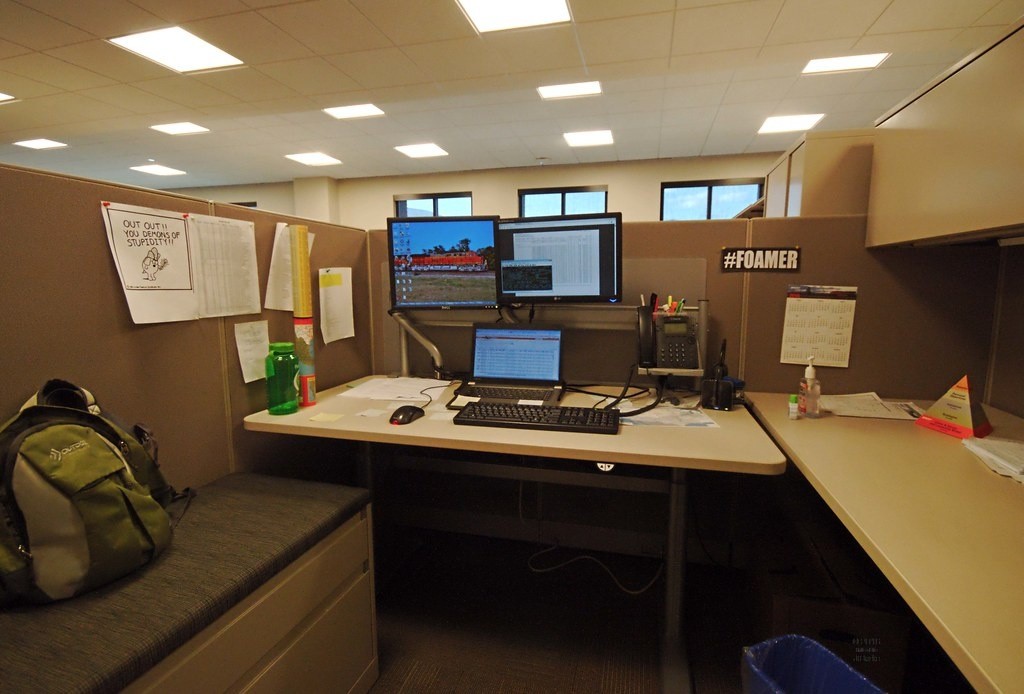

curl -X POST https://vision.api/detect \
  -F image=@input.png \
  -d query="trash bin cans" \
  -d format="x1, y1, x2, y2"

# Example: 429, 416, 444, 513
741, 631, 884, 694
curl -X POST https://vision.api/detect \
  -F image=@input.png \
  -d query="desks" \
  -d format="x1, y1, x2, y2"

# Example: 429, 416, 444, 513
740, 392, 1023, 694
241, 374, 787, 694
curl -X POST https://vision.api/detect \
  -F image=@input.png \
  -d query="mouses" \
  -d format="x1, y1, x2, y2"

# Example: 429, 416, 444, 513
389, 405, 425, 425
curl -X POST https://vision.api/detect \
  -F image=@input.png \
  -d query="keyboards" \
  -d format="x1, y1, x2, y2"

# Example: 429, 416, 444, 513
453, 401, 620, 434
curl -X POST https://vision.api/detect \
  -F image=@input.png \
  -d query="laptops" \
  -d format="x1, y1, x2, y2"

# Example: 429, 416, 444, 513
446, 322, 566, 410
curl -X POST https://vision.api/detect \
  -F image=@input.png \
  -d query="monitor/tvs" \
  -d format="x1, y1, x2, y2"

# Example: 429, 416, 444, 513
497, 211, 622, 304
387, 215, 502, 311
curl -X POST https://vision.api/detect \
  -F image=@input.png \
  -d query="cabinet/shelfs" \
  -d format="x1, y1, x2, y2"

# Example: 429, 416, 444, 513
763, 127, 875, 218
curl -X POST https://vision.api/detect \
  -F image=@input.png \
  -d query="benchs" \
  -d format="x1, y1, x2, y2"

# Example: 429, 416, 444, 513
0, 474, 381, 693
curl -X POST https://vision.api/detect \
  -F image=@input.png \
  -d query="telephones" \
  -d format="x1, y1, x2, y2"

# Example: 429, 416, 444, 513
638, 306, 700, 370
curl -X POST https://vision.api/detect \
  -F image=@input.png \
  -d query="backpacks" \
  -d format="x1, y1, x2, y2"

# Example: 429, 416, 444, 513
0, 378, 198, 607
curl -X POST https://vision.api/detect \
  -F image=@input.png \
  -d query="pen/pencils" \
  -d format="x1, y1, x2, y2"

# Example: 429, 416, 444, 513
640, 293, 686, 313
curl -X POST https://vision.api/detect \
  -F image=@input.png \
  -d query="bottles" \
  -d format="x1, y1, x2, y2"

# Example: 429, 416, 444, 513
265, 342, 299, 415
789, 394, 798, 420
